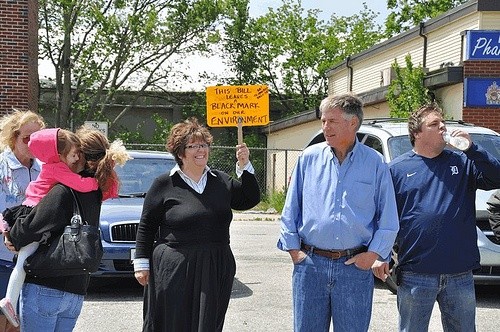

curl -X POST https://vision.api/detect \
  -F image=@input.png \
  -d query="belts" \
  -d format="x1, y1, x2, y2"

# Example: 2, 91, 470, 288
301, 243, 368, 259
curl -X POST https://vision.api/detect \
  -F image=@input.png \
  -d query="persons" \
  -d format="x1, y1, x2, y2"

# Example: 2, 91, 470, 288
134, 118, 261, 331
4, 125, 134, 331
0, 107, 46, 331
277, 91, 400, 331
388, 102, 499, 331
0, 127, 100, 327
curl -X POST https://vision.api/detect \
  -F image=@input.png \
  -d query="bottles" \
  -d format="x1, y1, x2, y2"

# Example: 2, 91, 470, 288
442, 130, 470, 150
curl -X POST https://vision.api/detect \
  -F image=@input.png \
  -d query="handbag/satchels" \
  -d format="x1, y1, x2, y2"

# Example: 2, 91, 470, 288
23, 188, 104, 276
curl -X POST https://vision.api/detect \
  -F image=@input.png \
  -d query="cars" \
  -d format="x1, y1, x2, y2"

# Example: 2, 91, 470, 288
89, 148, 177, 279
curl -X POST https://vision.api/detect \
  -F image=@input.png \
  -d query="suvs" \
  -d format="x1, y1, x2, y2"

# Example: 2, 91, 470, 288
302, 116, 500, 294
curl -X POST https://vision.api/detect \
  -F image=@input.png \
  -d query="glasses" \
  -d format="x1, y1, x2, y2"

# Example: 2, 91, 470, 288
185, 144, 209, 150
14, 132, 30, 144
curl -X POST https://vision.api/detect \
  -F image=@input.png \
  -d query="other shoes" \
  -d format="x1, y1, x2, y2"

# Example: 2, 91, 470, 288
0, 297, 19, 327
